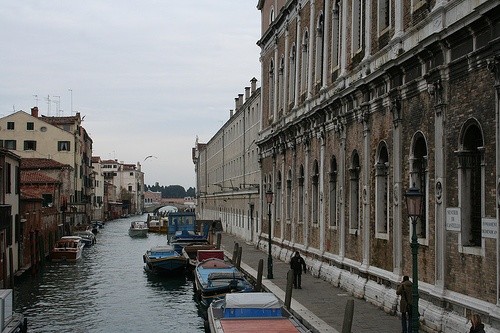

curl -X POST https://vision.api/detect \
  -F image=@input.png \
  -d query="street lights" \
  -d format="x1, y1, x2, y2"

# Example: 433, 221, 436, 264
265, 187, 275, 279
402, 182, 426, 333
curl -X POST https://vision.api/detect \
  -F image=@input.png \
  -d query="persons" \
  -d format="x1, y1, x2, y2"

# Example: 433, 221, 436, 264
290, 251, 306, 289
396, 276, 412, 333
469, 314, 485, 333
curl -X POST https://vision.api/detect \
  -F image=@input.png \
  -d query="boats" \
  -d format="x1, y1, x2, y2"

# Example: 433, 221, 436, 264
207, 291, 313, 333
142, 245, 190, 276
192, 258, 256, 310
68, 219, 105, 247
50, 236, 86, 262
130, 210, 229, 274
